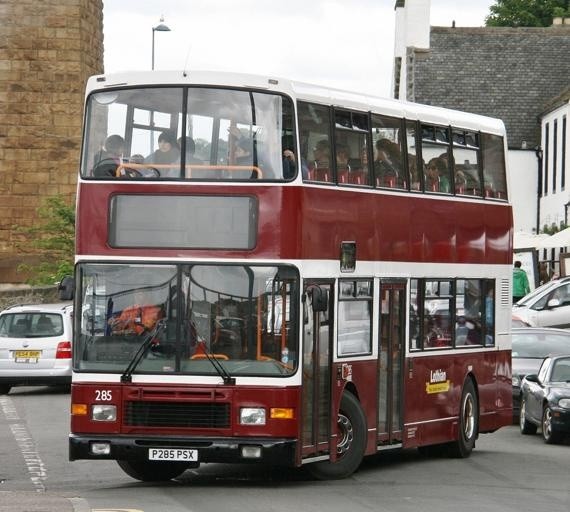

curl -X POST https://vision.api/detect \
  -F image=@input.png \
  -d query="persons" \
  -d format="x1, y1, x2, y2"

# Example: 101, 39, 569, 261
211, 286, 249, 354
513, 259, 532, 305
104, 272, 165, 339
306, 138, 482, 197
95, 124, 215, 181
226, 125, 310, 180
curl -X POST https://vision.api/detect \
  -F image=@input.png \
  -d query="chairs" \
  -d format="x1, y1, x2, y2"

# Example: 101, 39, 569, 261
7, 317, 55, 339
304, 156, 508, 205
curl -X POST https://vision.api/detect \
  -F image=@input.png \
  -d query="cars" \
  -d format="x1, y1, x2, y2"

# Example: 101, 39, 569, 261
84, 281, 105, 302
417, 275, 569, 450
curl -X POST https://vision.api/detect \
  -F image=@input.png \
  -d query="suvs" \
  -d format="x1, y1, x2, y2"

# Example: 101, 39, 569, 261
0, 301, 105, 401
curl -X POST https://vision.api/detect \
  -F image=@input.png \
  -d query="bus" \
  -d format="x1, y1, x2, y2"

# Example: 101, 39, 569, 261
64, 68, 519, 487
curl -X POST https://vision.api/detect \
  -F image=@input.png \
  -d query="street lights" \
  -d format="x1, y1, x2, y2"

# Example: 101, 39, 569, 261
148, 10, 172, 153
522, 140, 542, 235
